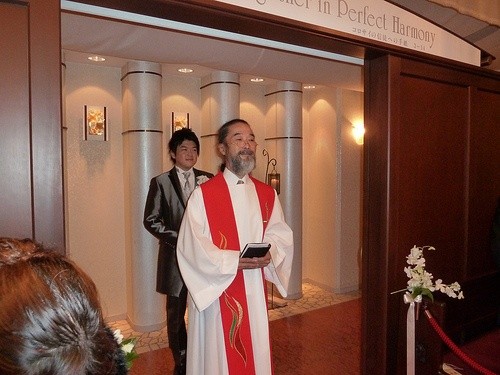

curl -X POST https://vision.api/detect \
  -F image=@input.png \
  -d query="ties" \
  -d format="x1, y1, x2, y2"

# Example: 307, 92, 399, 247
175, 168, 191, 199
237, 180, 245, 184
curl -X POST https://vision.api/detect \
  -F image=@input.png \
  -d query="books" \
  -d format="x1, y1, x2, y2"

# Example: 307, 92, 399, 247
240, 243, 271, 258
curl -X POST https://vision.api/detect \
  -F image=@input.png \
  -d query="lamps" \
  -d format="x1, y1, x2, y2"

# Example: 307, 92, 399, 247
83, 104, 109, 143
170, 111, 189, 137
262, 149, 281, 196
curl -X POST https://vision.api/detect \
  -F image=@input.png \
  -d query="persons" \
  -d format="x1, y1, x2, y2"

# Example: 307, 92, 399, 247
176, 119, 294, 375
0, 236, 139, 375
144, 129, 214, 375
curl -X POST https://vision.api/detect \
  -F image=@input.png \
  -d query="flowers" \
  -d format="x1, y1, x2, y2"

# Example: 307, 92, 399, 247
403, 244, 464, 304
113, 328, 141, 370
